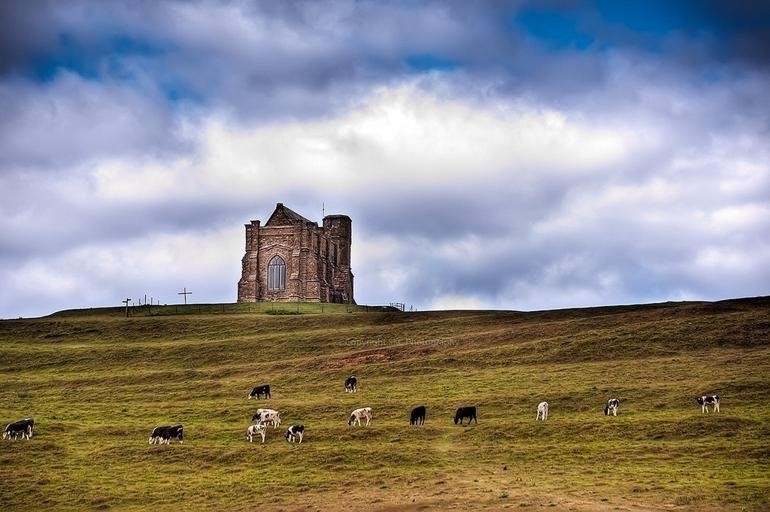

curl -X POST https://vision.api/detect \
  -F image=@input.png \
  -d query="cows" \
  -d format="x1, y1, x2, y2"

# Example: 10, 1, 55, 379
2, 418, 35, 442
245, 407, 281, 443
603, 398, 620, 418
408, 404, 427, 427
696, 393, 720, 413
344, 374, 358, 393
285, 424, 305, 444
536, 401, 549, 421
348, 406, 374, 427
453, 405, 478, 425
149, 424, 184, 446
248, 382, 271, 401
